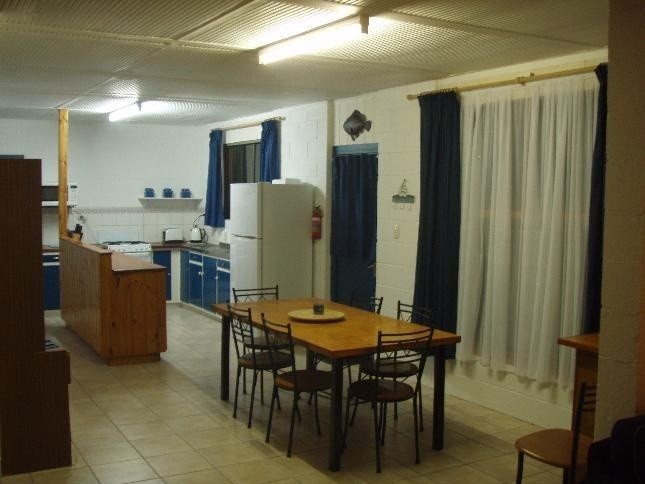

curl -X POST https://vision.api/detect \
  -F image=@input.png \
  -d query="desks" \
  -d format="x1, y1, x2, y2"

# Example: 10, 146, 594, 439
556, 333, 599, 435
211, 299, 462, 472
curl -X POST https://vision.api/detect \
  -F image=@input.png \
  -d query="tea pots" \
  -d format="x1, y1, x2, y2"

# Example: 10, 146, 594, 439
189, 225, 205, 243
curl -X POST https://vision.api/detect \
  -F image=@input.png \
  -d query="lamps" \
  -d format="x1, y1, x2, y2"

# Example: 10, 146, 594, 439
108, 100, 142, 123
255, 12, 370, 67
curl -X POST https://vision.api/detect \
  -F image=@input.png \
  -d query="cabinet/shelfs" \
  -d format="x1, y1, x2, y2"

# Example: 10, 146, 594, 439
188, 251, 230, 319
179, 250, 189, 305
0, 157, 73, 476
59, 237, 166, 367
152, 251, 172, 301
42, 266, 59, 308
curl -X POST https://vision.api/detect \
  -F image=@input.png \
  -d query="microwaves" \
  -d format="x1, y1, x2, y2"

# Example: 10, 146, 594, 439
42, 183, 77, 207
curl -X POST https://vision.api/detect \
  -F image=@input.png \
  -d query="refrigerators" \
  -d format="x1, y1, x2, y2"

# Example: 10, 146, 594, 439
228, 183, 315, 345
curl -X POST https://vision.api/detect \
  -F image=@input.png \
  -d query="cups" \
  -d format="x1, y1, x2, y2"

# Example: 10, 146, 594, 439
314, 305, 324, 314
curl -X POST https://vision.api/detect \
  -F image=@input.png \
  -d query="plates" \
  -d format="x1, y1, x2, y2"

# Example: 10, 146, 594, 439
185, 241, 205, 246
288, 309, 345, 322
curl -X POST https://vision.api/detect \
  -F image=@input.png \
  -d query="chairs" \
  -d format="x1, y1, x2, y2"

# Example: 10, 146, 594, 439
515, 382, 598, 483
233, 287, 279, 402
345, 325, 435, 472
258, 312, 331, 458
307, 296, 384, 405
224, 307, 294, 428
350, 302, 435, 432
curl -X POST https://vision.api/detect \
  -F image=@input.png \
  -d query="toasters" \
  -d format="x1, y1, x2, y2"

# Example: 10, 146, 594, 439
163, 228, 184, 243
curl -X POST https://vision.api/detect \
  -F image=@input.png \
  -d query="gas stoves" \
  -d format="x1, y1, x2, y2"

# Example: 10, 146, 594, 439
104, 240, 148, 250
